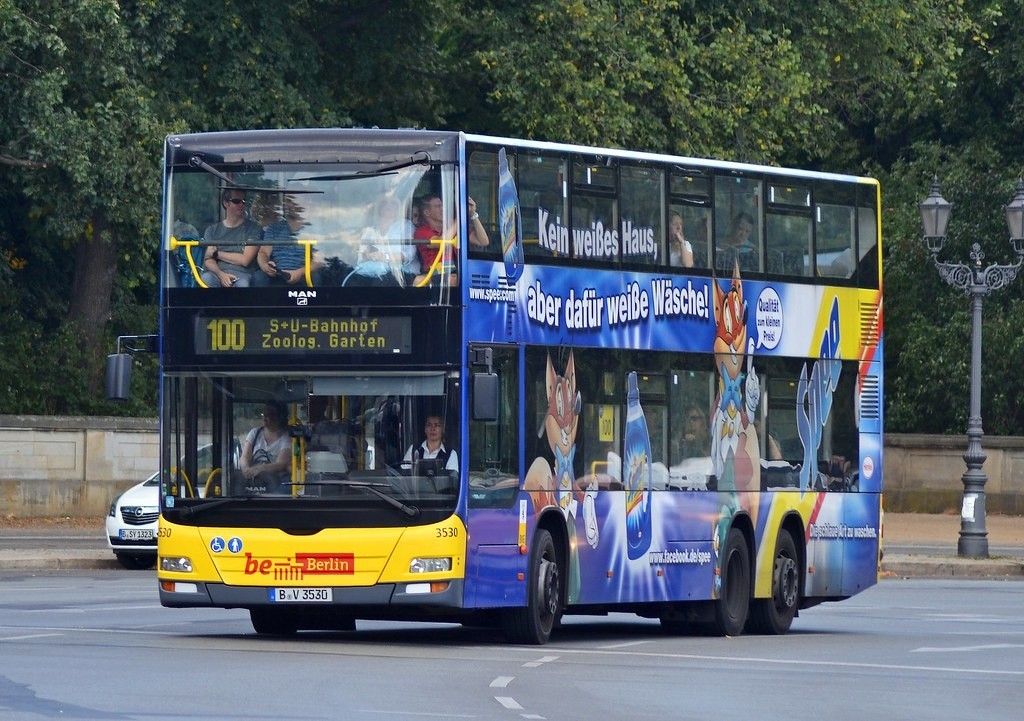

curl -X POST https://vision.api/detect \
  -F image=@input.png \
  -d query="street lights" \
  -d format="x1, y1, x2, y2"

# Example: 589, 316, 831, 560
919, 171, 1024, 559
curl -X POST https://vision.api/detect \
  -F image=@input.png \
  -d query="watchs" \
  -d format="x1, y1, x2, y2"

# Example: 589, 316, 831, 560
681, 241, 687, 245
471, 213, 480, 221
384, 253, 391, 262
211, 250, 220, 257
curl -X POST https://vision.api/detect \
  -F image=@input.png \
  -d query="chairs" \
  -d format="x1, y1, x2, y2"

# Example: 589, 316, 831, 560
470, 222, 804, 276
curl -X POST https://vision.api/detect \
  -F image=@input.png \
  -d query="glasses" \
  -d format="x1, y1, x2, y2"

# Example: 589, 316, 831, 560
259, 410, 274, 418
228, 198, 246, 204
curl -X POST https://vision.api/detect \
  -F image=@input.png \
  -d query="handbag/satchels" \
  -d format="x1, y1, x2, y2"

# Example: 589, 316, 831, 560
249, 449, 279, 491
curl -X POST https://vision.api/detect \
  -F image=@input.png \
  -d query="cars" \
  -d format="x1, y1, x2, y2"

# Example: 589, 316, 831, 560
105, 441, 239, 570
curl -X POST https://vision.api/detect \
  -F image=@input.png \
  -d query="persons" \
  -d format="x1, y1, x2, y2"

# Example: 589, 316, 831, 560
194, 181, 490, 287
240, 394, 459, 498
655, 210, 760, 271
639, 406, 783, 463
173, 202, 200, 244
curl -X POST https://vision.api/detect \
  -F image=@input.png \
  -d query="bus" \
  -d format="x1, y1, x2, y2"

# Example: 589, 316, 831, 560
104, 128, 887, 649
